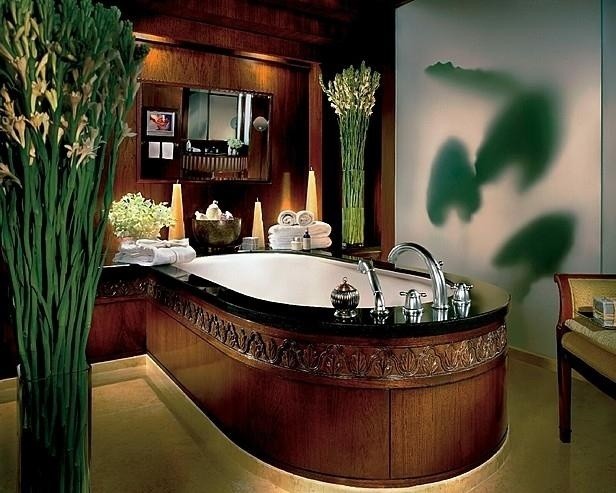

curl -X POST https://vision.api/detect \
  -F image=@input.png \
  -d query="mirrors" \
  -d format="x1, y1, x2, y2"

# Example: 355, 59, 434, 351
135, 77, 274, 186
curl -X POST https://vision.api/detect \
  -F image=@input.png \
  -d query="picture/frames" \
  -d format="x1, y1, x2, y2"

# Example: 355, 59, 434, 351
143, 104, 178, 138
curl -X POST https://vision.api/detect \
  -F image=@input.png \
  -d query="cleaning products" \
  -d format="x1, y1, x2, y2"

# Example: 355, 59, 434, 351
303, 230, 311, 251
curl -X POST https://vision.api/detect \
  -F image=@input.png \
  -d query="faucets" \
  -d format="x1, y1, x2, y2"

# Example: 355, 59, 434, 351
387, 242, 448, 310
357, 257, 385, 311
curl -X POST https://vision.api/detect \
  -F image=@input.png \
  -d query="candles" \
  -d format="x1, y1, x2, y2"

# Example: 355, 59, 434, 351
169, 180, 186, 241
253, 197, 266, 249
306, 166, 319, 222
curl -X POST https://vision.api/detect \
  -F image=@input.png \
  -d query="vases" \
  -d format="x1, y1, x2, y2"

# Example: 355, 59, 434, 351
341, 170, 364, 242
15, 362, 92, 492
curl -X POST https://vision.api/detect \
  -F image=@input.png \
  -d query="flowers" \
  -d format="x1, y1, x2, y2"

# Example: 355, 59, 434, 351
314, 59, 380, 245
1, 1, 147, 493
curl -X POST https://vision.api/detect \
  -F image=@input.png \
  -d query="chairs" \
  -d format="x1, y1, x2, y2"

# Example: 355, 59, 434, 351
553, 274, 615, 442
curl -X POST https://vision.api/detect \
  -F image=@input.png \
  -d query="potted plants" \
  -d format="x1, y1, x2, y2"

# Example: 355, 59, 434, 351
110, 191, 173, 245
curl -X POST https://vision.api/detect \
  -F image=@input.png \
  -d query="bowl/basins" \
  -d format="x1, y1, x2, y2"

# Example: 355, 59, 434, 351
191, 217, 242, 250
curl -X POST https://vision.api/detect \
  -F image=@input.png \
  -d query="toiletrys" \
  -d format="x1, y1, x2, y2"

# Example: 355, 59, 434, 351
302, 228, 312, 251
290, 236, 304, 250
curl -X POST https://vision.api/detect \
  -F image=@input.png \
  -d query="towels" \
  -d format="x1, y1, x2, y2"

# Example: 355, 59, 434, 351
268, 210, 333, 252
112, 238, 197, 266
